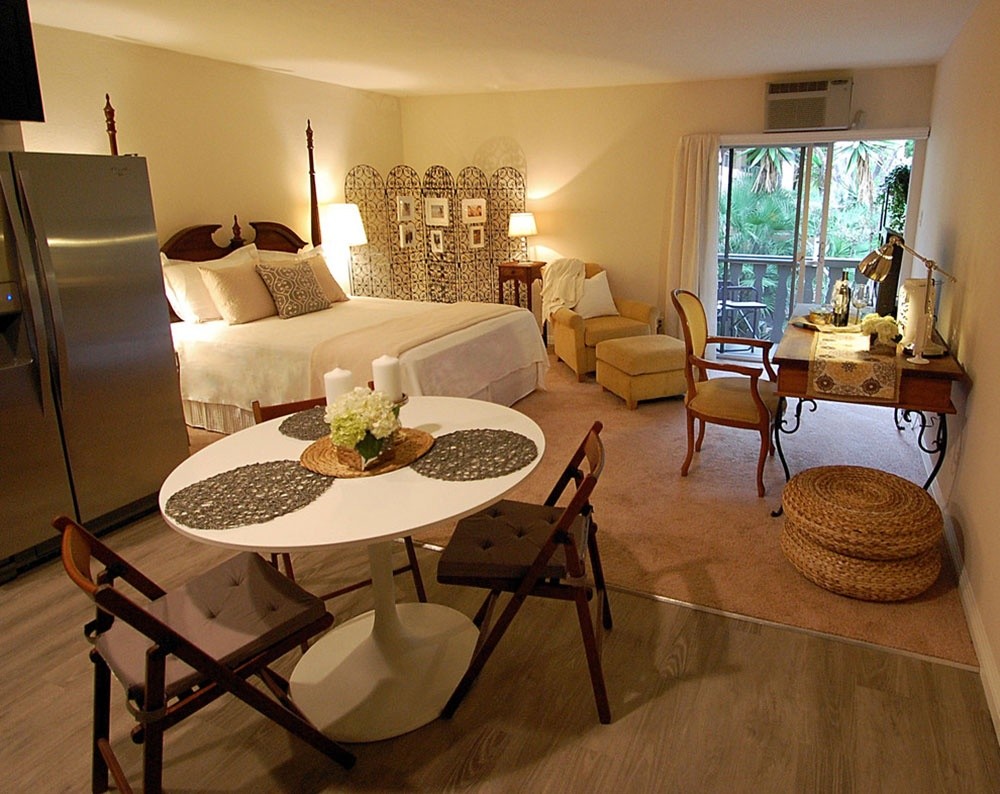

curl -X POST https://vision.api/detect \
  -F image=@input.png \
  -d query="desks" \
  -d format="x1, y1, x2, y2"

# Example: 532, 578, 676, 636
495, 261, 546, 315
159, 394, 542, 743
772, 313, 972, 524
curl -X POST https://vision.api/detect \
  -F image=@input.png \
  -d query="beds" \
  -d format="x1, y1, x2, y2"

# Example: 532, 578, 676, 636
104, 92, 549, 437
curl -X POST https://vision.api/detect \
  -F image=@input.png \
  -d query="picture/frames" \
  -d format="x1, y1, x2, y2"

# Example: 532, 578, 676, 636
397, 195, 416, 222
462, 198, 486, 224
399, 225, 414, 246
425, 196, 449, 226
469, 228, 485, 248
429, 231, 444, 253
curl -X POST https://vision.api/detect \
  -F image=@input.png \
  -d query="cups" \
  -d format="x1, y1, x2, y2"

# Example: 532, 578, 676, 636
809, 306, 831, 325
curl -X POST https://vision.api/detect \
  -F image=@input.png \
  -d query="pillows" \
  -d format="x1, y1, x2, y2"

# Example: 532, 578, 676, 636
197, 261, 278, 324
258, 263, 331, 319
298, 253, 349, 304
153, 244, 257, 325
260, 245, 314, 264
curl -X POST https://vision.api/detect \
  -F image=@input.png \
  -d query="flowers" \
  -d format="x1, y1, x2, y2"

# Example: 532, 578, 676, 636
328, 394, 400, 462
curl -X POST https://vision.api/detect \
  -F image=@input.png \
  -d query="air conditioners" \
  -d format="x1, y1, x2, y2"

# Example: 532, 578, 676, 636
762, 76, 854, 133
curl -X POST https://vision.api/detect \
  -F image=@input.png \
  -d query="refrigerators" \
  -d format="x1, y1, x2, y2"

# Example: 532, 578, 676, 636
0, 151, 190, 586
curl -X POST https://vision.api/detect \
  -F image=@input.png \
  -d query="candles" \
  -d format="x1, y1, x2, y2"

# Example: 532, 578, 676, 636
371, 354, 401, 407
322, 368, 355, 411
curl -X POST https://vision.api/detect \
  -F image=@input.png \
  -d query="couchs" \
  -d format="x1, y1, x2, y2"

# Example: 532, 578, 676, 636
542, 260, 658, 381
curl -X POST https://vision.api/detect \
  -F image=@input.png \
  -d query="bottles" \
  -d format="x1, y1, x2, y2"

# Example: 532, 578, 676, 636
834, 272, 851, 326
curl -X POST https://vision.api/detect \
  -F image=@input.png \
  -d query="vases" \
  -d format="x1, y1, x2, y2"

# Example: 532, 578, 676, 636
335, 438, 397, 473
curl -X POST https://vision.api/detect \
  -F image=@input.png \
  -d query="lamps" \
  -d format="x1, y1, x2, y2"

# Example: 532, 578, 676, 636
859, 236, 959, 365
507, 211, 537, 260
315, 202, 367, 292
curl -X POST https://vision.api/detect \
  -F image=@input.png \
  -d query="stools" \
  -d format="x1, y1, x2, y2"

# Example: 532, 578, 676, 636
780, 468, 944, 604
593, 333, 691, 411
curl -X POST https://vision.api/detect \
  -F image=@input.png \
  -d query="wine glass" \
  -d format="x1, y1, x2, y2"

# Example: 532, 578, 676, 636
851, 283, 870, 324
831, 285, 840, 313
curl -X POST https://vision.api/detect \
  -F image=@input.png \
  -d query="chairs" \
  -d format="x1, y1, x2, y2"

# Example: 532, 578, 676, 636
672, 288, 788, 499
437, 422, 615, 726
51, 515, 358, 794
249, 379, 424, 655
717, 286, 767, 354
